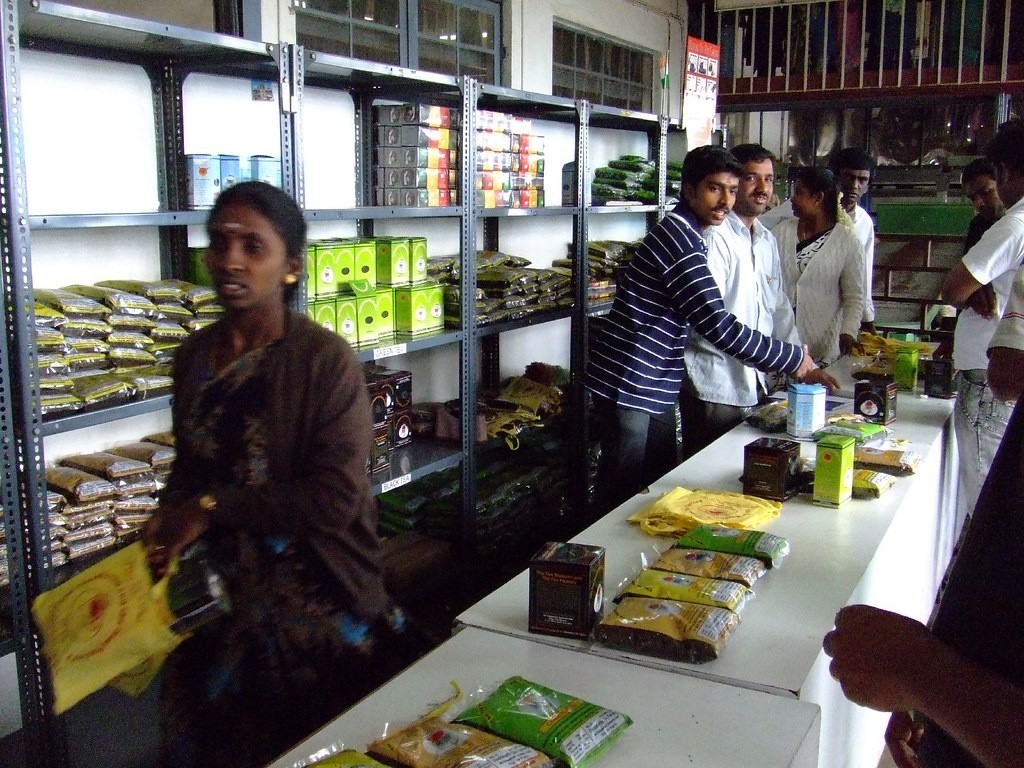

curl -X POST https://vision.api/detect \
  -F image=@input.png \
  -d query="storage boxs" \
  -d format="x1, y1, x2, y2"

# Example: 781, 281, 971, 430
374, 101, 545, 208
328, 242, 355, 297
395, 289, 427, 336
362, 362, 415, 476
376, 239, 410, 288
854, 380, 899, 427
561, 161, 578, 207
307, 245, 316, 302
309, 300, 336, 332
812, 434, 855, 510
924, 360, 958, 399
529, 542, 606, 642
786, 384, 826, 442
355, 247, 376, 290
420, 282, 444, 332
742, 437, 801, 503
310, 244, 336, 301
374, 287, 394, 340
893, 347, 919, 392
347, 279, 379, 347
398, 236, 428, 286
184, 150, 281, 290
325, 295, 358, 347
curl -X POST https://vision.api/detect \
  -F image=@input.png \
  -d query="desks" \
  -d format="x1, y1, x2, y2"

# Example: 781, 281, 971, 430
265, 625, 822, 767
448, 343, 954, 768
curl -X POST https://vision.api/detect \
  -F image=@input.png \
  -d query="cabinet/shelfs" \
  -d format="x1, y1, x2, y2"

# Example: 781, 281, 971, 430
288, 44, 473, 599
582, 100, 670, 370
0, 0, 295, 768
469, 78, 584, 540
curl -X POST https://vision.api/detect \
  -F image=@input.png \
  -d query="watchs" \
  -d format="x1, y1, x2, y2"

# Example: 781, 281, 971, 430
199, 492, 217, 512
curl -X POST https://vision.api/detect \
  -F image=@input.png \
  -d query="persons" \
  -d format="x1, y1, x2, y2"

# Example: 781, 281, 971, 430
823, 387, 1024, 768
140, 183, 391, 768
584, 143, 877, 517
941, 116, 1024, 522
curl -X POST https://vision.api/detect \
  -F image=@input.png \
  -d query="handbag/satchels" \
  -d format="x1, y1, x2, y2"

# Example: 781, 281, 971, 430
483, 409, 544, 453
29, 537, 192, 719
